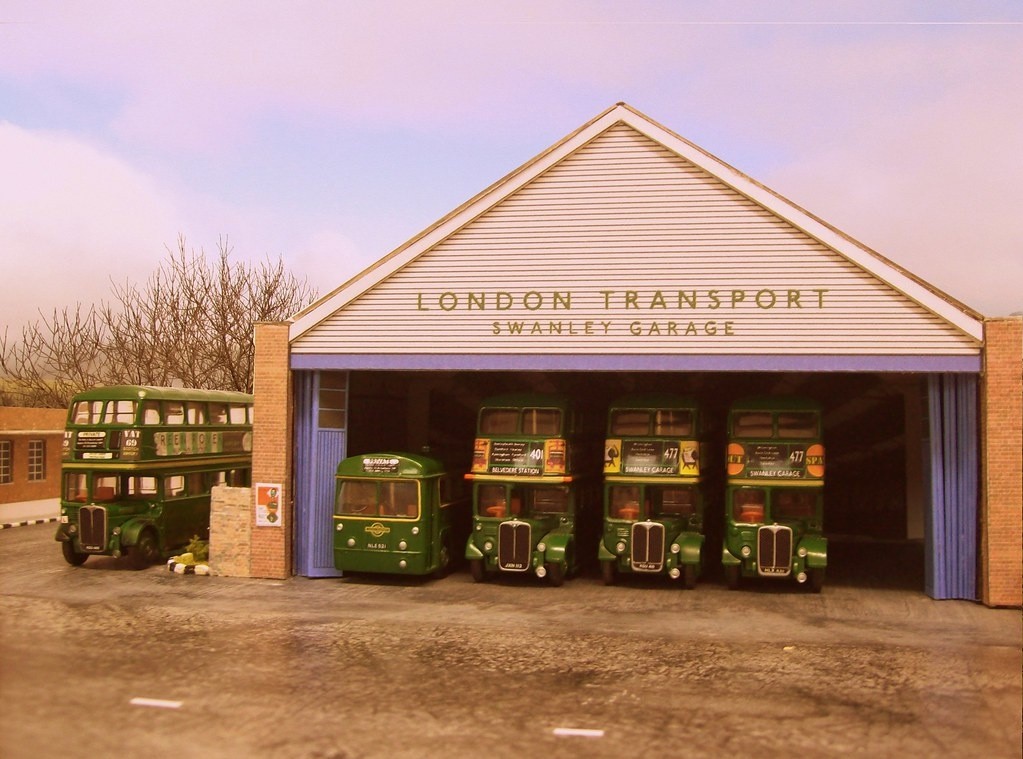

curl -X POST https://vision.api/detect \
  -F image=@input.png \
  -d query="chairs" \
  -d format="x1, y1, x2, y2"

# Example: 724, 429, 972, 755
742, 504, 764, 516
624, 501, 649, 517
496, 498, 521, 516
96, 487, 114, 499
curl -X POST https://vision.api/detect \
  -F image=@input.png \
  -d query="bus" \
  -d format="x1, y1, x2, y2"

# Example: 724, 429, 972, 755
596, 391, 720, 591
332, 451, 471, 580
722, 395, 827, 593
56, 384, 254, 570
464, 393, 600, 588
